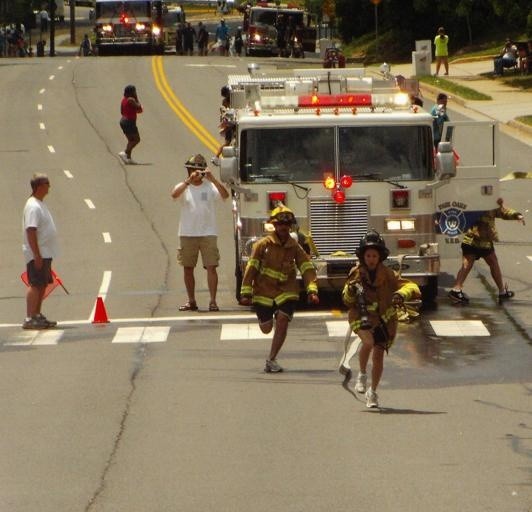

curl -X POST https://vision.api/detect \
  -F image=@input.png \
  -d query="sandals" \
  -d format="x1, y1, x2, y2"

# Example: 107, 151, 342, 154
209, 304, 219, 311
179, 302, 198, 311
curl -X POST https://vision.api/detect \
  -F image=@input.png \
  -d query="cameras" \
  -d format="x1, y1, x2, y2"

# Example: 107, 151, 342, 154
196, 170, 206, 177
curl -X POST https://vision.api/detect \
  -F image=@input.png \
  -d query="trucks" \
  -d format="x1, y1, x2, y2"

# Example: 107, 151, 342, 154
244, 1, 316, 57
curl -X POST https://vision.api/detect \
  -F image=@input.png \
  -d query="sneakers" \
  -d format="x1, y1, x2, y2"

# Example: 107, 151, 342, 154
448, 290, 469, 304
119, 152, 137, 164
365, 386, 378, 407
499, 291, 514, 299
22, 315, 57, 329
355, 371, 367, 393
264, 360, 283, 373
211, 157, 220, 166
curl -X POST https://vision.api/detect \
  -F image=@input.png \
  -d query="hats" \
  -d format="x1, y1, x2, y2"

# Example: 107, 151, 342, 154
184, 154, 208, 169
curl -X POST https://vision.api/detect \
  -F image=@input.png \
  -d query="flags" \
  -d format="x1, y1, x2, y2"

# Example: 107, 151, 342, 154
19, 270, 62, 298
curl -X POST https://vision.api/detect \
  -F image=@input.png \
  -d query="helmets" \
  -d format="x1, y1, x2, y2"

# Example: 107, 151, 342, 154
355, 231, 390, 263
268, 205, 295, 224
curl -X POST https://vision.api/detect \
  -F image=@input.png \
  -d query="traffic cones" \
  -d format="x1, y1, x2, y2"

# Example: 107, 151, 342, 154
92, 296, 110, 324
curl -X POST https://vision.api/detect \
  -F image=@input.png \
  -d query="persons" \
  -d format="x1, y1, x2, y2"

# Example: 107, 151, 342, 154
432, 93, 449, 145
239, 201, 320, 373
434, 27, 450, 74
210, 86, 231, 165
494, 38, 519, 76
448, 202, 525, 303
171, 153, 229, 311
23, 172, 57, 329
342, 230, 421, 408
1, 7, 96, 57
162, 1, 251, 58
119, 85, 142, 164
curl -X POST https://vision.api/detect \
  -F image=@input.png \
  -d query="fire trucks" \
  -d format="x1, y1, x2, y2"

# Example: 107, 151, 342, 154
90, 0, 186, 55
219, 68, 500, 305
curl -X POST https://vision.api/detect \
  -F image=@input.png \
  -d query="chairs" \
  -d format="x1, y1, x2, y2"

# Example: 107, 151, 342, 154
493, 42, 518, 72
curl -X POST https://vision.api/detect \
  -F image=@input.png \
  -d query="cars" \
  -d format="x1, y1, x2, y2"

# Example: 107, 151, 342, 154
245, 24, 279, 56
